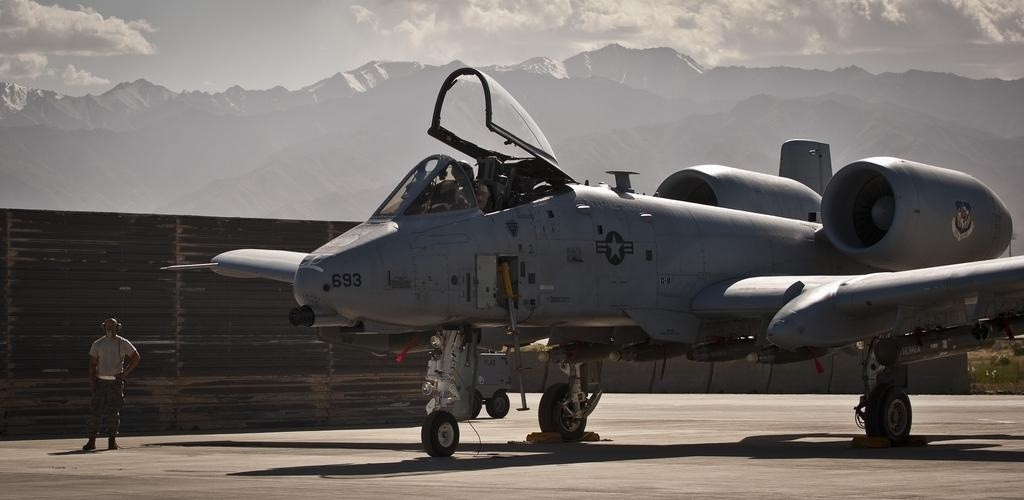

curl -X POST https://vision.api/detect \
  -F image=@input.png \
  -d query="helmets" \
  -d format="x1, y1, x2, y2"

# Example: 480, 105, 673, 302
450, 160, 474, 183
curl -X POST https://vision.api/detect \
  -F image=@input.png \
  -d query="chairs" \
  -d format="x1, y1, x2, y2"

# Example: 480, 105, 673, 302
461, 156, 509, 211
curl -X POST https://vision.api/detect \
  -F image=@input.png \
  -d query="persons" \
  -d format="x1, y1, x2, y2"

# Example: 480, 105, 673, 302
467, 180, 490, 212
80, 317, 141, 450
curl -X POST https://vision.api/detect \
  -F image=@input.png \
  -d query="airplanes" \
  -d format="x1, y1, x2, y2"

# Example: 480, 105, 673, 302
159, 68, 1024, 457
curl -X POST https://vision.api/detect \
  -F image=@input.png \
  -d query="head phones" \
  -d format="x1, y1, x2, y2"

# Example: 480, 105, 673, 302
101, 322, 122, 330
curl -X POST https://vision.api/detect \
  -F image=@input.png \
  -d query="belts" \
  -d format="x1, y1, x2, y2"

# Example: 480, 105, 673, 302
95, 374, 118, 381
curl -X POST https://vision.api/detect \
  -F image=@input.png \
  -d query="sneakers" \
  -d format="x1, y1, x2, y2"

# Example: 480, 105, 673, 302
82, 437, 96, 451
108, 436, 118, 450
